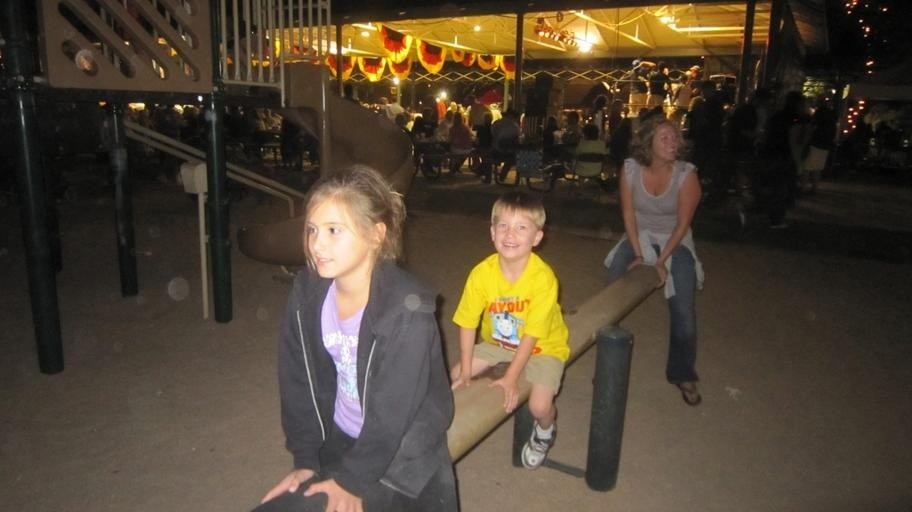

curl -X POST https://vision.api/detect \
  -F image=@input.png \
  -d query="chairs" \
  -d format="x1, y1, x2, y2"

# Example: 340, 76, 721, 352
402, 142, 608, 194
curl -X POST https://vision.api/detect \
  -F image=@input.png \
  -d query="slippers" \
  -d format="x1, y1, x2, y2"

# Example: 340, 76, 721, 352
677, 383, 701, 405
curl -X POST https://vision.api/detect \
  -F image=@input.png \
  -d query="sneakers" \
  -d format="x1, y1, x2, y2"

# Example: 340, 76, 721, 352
521, 419, 557, 470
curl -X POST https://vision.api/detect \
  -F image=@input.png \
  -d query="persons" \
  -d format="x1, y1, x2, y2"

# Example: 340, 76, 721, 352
260, 162, 460, 511
449, 187, 572, 471
223, 11, 279, 83
99, 64, 912, 229
602, 110, 705, 407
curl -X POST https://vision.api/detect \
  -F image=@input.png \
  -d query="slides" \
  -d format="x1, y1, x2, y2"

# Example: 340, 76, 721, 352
238, 62, 416, 266
